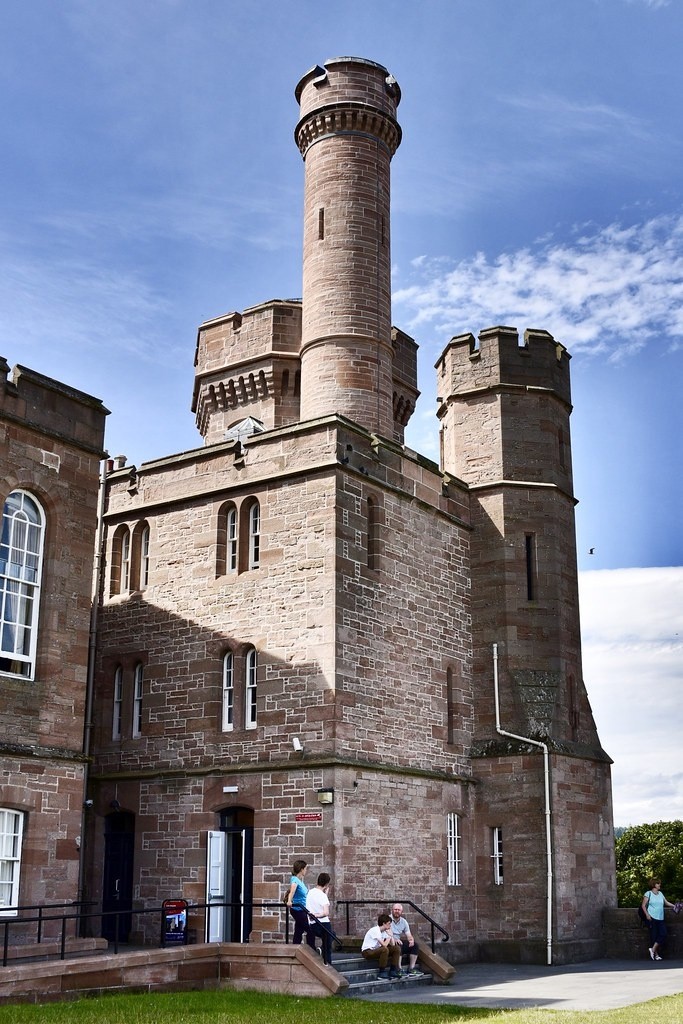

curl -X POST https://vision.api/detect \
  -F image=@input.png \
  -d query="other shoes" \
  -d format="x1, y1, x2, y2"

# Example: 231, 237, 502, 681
649, 948, 655, 961
655, 954, 662, 961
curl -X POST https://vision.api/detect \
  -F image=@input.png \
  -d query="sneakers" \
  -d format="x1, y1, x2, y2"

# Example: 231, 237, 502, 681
389, 968, 401, 978
408, 968, 424, 976
377, 969, 390, 980
396, 968, 408, 976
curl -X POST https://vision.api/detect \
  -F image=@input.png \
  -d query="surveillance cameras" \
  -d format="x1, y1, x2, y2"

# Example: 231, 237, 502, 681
87, 800, 92, 807
292, 738, 302, 752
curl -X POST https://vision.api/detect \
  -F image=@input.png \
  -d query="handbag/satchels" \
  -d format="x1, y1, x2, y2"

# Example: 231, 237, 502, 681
283, 887, 292, 904
638, 891, 650, 921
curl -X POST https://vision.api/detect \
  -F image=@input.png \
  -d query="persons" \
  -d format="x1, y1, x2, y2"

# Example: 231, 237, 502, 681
306, 873, 332, 965
642, 879, 675, 960
287, 860, 308, 944
361, 903, 424, 980
